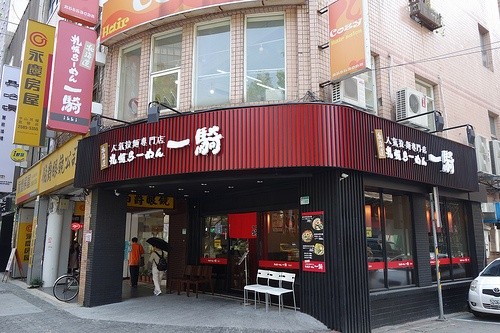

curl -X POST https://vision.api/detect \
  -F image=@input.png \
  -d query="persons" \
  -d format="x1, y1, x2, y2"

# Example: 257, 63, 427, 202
149, 246, 168, 295
128, 237, 145, 289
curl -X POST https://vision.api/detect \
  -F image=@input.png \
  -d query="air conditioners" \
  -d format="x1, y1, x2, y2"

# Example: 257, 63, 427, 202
395, 87, 428, 128
331, 76, 366, 109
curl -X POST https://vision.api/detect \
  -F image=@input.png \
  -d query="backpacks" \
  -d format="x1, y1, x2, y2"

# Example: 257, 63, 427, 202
153, 251, 168, 271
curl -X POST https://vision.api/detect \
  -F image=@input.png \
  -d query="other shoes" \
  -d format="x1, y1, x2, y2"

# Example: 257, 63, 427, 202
153, 291, 156, 294
156, 291, 162, 296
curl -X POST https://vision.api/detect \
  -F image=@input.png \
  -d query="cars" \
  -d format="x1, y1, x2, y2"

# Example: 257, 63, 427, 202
467, 257, 500, 319
369, 238, 403, 264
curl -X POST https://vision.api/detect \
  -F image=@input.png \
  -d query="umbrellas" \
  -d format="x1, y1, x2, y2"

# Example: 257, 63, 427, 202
145, 237, 170, 253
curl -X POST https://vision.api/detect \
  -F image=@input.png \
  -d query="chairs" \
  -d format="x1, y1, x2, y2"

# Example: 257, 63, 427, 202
243, 269, 296, 317
169, 265, 214, 299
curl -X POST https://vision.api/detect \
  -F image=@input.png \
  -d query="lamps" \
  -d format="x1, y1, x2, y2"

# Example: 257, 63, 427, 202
89, 115, 133, 135
145, 100, 186, 125
429, 123, 475, 146
394, 110, 444, 133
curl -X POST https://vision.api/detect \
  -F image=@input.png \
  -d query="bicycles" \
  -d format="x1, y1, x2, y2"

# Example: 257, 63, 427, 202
52, 268, 79, 302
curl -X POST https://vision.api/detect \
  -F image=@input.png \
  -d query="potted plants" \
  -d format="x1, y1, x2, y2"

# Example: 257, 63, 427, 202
139, 270, 152, 283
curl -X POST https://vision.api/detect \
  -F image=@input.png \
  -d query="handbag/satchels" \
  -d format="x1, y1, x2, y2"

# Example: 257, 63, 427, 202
140, 256, 145, 267
